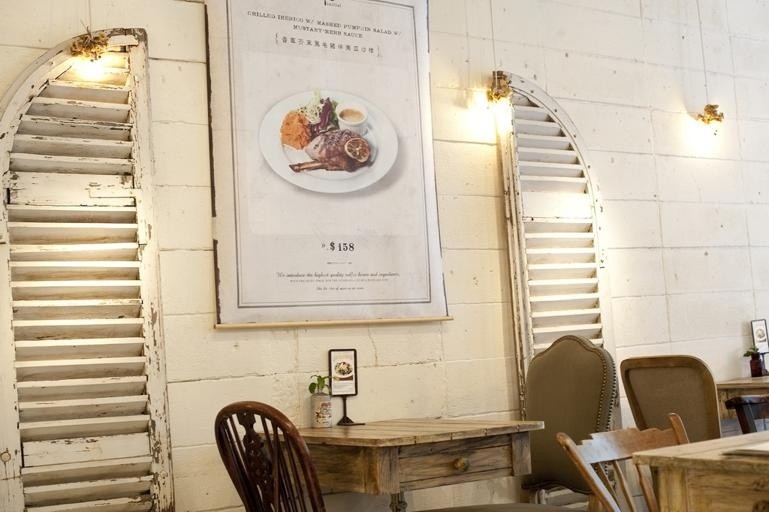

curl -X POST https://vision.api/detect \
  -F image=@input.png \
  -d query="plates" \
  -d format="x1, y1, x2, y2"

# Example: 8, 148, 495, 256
333, 360, 354, 378
257, 87, 398, 194
754, 325, 766, 341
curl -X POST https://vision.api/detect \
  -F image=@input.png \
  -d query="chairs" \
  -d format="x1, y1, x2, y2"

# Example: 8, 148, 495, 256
619, 349, 727, 445
205, 396, 328, 512
518, 333, 617, 512
552, 409, 690, 512
722, 392, 768, 438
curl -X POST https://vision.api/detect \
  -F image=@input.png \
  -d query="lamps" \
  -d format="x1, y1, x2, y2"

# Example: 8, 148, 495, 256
469, 0, 528, 132
677, 1, 731, 142
60, 0, 112, 66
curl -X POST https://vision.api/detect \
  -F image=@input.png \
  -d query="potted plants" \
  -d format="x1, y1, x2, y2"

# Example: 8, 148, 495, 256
303, 372, 338, 429
743, 346, 768, 377
